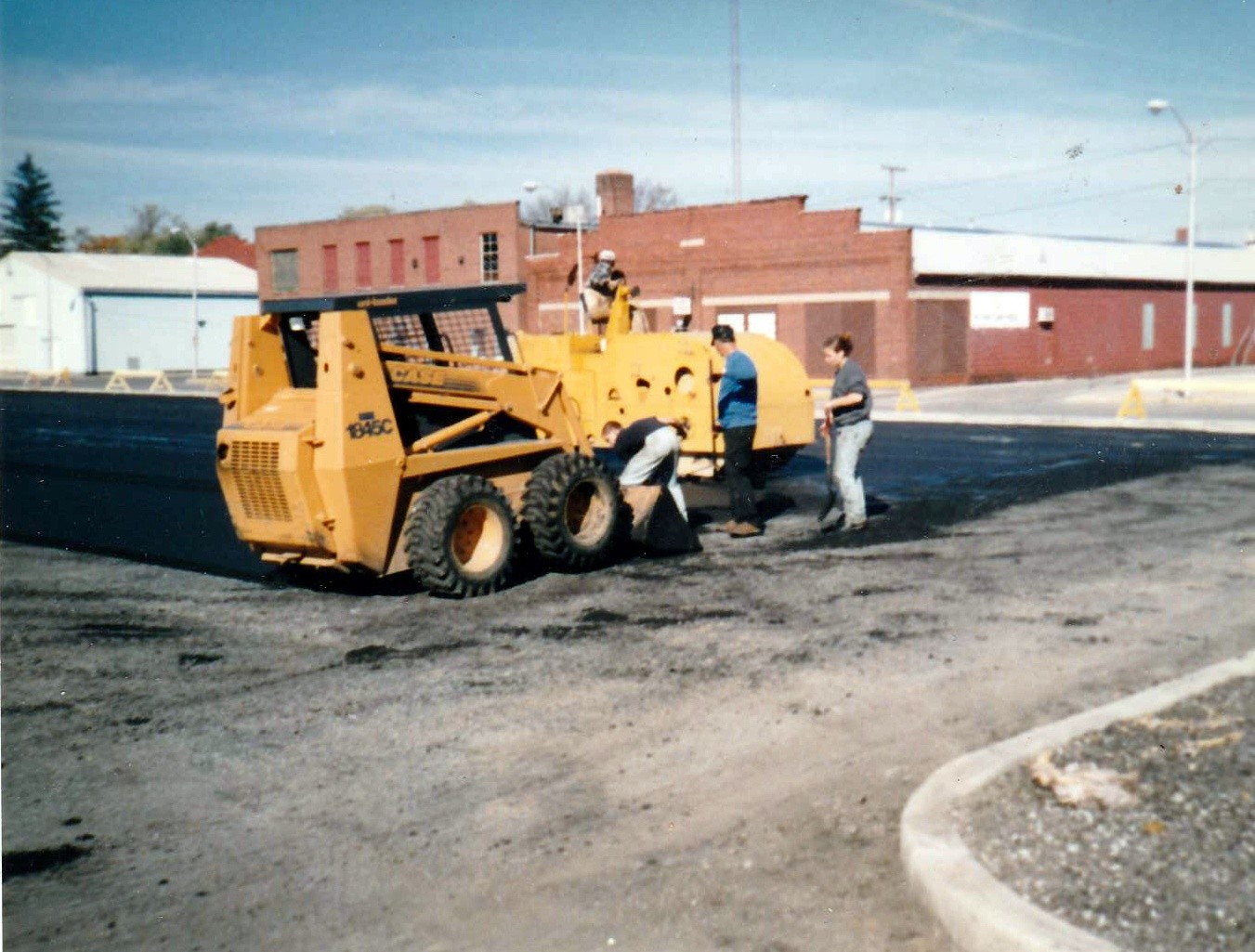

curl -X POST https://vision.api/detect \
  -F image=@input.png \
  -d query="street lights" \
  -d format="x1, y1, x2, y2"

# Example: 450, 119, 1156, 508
1145, 98, 1198, 399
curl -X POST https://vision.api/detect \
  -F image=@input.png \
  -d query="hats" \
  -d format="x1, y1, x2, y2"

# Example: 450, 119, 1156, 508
711, 325, 733, 345
611, 271, 624, 280
599, 249, 616, 261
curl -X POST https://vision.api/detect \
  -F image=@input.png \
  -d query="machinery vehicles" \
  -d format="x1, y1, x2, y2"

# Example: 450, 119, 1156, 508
210, 277, 817, 599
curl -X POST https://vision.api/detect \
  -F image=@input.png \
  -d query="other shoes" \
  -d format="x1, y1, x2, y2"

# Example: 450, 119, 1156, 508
841, 519, 868, 533
717, 519, 738, 532
687, 543, 704, 552
729, 521, 762, 535
640, 550, 657, 557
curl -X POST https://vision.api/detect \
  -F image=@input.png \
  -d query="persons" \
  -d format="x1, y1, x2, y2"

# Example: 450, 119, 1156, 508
600, 416, 703, 565
389, 321, 432, 363
809, 332, 873, 531
470, 328, 486, 357
589, 250, 650, 333
710, 325, 766, 538
